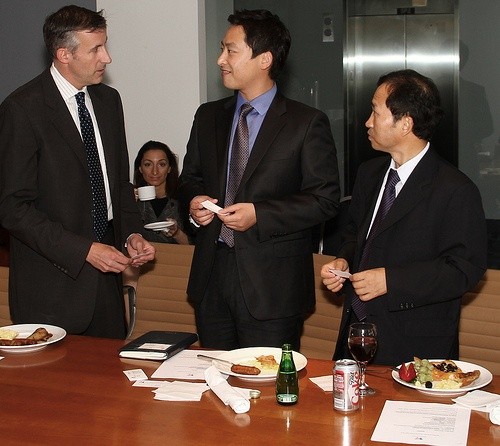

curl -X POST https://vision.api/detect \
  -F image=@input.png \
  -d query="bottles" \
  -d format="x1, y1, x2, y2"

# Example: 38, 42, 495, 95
276, 345, 299, 405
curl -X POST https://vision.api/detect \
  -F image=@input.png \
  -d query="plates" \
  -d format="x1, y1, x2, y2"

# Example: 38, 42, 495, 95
212, 347, 307, 381
0, 324, 66, 352
392, 360, 493, 396
144, 222, 175, 228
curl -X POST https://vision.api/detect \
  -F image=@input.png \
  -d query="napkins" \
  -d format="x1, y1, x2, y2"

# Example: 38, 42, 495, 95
308, 375, 369, 394
154, 381, 207, 402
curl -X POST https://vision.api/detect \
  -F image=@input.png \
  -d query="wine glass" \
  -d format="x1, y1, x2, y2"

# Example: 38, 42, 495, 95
347, 322, 378, 397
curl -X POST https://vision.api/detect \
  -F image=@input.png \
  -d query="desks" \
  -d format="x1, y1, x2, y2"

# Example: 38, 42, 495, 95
0, 335, 500, 446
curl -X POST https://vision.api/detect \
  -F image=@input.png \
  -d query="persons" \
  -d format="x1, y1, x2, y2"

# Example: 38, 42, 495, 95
132, 140, 192, 245
171, 9, 342, 353
321, 68, 489, 368
0, 4, 155, 342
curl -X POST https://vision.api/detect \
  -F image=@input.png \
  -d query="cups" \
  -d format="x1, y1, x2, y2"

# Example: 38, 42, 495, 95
135, 185, 156, 201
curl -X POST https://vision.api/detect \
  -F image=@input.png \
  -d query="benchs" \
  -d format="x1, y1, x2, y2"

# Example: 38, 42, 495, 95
0, 242, 500, 375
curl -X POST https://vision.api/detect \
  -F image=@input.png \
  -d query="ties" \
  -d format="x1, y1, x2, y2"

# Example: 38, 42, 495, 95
350, 168, 401, 322
74, 92, 108, 243
220, 103, 254, 248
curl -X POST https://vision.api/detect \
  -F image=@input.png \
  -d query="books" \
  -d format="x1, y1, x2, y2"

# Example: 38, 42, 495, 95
118, 331, 200, 361
